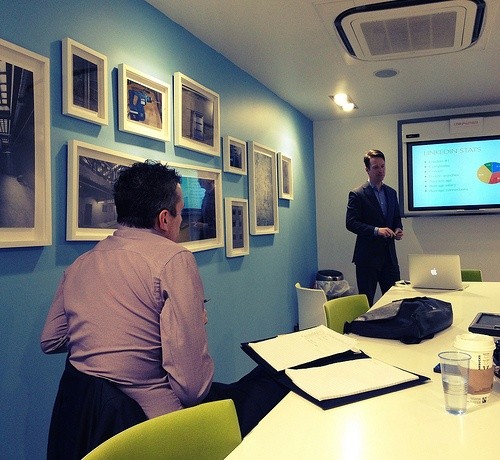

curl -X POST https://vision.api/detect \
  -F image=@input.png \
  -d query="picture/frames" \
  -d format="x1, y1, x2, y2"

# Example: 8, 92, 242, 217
222, 136, 248, 176
66, 139, 151, 241
0, 38, 52, 248
174, 72, 221, 158
118, 62, 171, 143
61, 36, 109, 126
247, 141, 279, 236
277, 151, 294, 201
225, 197, 250, 257
156, 160, 225, 253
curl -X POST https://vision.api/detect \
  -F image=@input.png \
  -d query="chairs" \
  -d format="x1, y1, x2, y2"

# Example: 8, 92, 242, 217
322, 294, 371, 335
80, 398, 242, 460
460, 269, 483, 282
295, 282, 328, 331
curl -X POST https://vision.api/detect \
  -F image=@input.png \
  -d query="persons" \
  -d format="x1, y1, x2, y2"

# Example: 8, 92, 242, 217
346, 150, 403, 308
39, 159, 289, 433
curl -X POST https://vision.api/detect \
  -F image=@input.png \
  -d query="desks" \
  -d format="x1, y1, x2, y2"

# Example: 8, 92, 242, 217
224, 279, 500, 460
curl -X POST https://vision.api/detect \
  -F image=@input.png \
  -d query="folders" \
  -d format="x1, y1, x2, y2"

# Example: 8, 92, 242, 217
240, 325, 431, 411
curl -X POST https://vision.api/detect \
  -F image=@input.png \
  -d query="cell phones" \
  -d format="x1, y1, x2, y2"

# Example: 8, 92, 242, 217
434, 363, 468, 375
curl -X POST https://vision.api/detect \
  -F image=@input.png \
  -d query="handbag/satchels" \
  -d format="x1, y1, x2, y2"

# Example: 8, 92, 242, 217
343, 296, 453, 344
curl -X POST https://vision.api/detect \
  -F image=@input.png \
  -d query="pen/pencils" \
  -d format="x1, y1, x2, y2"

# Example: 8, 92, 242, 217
204, 298, 212, 302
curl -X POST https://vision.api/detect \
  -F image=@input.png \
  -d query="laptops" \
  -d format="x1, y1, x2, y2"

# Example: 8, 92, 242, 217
408, 253, 470, 291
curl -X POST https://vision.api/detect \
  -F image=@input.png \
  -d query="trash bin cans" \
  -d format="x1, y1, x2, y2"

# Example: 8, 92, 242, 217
316, 269, 343, 300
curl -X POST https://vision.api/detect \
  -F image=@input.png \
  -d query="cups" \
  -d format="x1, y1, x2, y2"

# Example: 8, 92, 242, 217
454, 334, 496, 404
437, 351, 470, 414
